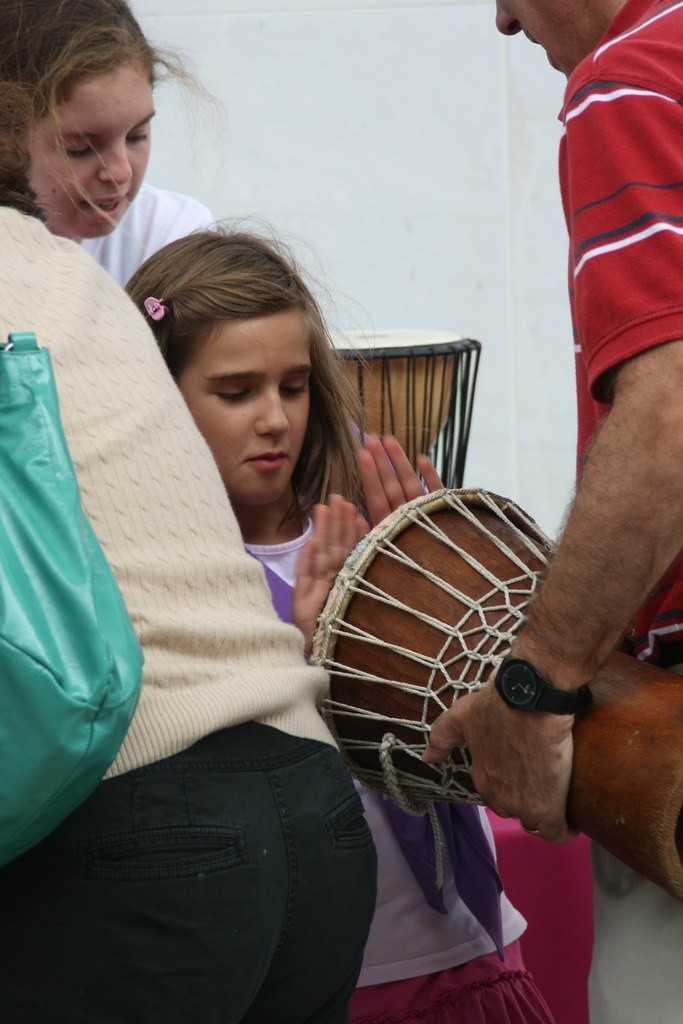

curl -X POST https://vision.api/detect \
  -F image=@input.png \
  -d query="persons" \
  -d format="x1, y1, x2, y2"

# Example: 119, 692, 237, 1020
0, 0, 683, 1024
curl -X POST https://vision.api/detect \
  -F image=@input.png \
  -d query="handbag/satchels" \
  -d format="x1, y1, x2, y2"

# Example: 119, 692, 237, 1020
0, 333, 145, 866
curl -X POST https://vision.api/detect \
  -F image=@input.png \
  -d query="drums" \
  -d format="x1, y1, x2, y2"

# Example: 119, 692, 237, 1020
327, 328, 485, 519
300, 486, 683, 913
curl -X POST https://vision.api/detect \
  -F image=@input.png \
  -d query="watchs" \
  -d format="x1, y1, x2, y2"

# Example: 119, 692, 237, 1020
494, 653, 594, 717
